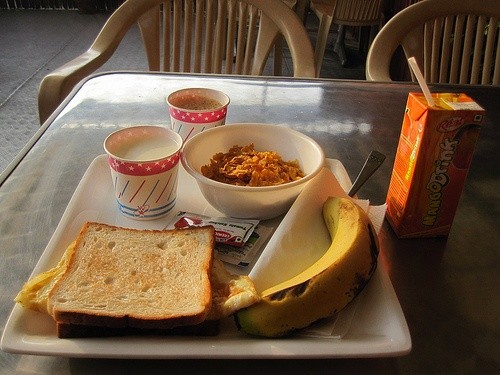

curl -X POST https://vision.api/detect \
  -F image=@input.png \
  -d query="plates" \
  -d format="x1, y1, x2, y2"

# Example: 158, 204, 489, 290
0, 154, 412, 357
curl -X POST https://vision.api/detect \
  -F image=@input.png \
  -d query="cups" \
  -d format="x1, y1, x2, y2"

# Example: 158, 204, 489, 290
166, 87, 231, 158
104, 124, 183, 220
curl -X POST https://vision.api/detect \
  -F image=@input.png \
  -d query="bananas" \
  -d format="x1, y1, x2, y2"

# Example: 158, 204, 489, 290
235, 196, 381, 339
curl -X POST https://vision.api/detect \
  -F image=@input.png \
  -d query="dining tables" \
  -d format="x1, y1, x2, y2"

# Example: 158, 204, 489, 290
0, 71, 500, 375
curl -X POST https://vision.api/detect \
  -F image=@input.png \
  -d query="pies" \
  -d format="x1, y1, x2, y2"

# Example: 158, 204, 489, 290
13, 240, 259, 323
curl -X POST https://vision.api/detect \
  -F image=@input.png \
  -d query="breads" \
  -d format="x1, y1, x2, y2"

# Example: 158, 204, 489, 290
48, 220, 216, 338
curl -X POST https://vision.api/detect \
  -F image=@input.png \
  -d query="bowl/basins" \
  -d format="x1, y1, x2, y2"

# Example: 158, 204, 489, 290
181, 123, 324, 220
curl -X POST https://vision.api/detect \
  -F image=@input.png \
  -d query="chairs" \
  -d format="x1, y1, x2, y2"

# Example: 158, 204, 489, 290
37, 0, 500, 126
